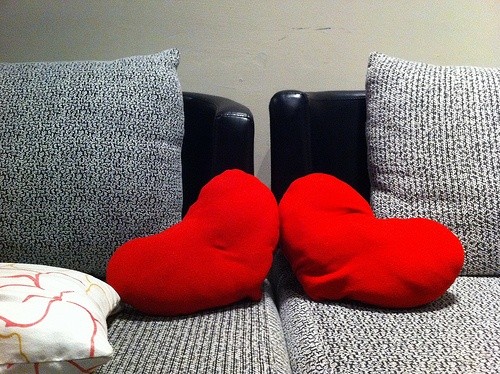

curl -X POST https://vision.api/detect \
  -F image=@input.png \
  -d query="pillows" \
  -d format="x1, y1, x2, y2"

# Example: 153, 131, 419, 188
0, 263, 120, 374
363, 49, 500, 279
105, 167, 280, 318
0, 45, 185, 284
276, 171, 466, 313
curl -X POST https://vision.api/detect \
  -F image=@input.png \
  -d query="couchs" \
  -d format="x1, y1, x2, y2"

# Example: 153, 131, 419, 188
268, 88, 500, 374
75, 89, 293, 374
